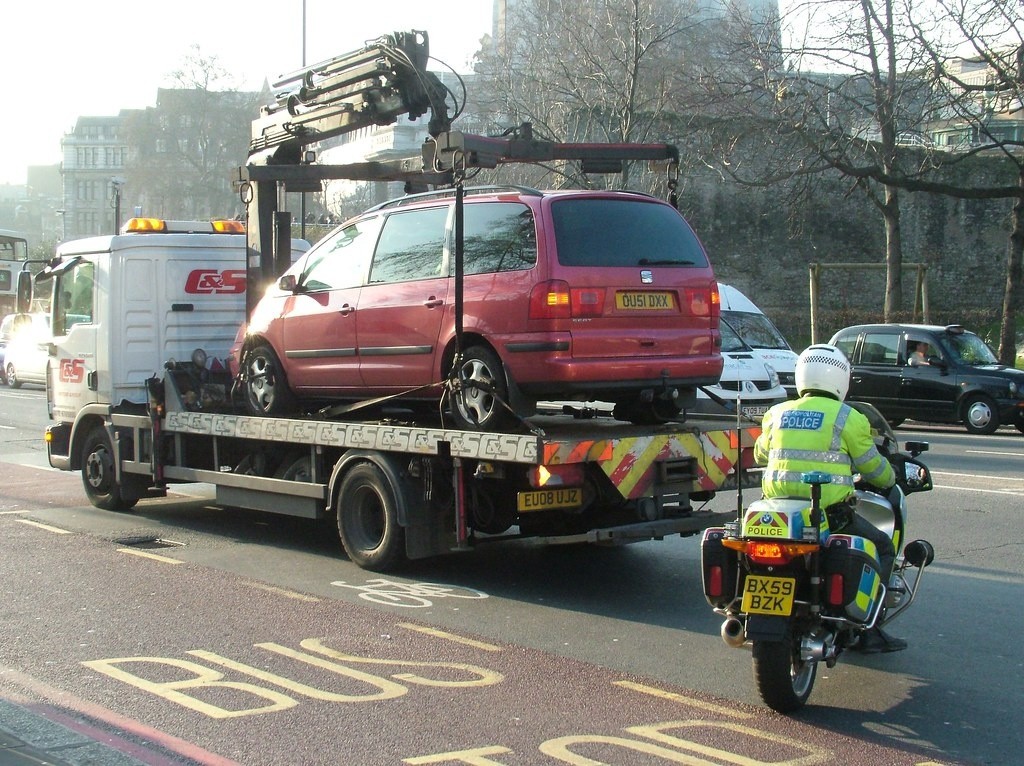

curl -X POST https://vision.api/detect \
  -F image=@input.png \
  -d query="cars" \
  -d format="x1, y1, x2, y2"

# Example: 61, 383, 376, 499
829, 323, 1024, 434
2, 336, 67, 389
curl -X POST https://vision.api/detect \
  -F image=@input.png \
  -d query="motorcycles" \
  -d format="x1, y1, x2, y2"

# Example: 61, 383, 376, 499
699, 397, 935, 713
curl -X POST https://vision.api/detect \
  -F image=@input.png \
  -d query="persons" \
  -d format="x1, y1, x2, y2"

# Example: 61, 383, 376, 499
754, 344, 908, 655
907, 342, 933, 368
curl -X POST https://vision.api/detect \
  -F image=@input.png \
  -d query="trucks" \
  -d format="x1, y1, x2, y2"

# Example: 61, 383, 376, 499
15, 215, 768, 573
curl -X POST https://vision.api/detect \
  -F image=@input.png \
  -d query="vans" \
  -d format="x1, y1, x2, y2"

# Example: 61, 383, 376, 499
535, 275, 801, 420
0, 229, 30, 326
0, 313, 92, 385
714, 282, 800, 401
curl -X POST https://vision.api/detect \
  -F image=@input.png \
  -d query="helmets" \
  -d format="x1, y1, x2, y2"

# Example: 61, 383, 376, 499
795, 343, 851, 401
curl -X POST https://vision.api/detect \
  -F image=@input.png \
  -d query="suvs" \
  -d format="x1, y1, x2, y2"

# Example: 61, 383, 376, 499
227, 182, 723, 430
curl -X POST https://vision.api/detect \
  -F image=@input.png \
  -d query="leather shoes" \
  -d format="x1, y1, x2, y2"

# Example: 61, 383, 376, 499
858, 627, 909, 655
